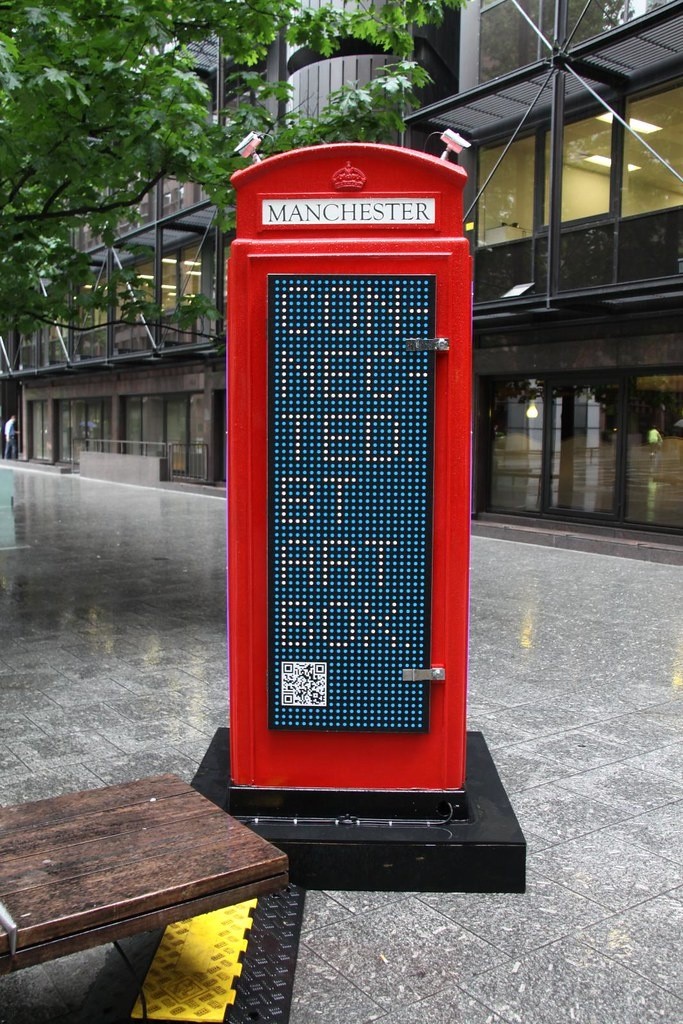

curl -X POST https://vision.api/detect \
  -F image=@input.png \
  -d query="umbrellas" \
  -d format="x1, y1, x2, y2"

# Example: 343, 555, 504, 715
78, 421, 98, 428
672, 419, 683, 427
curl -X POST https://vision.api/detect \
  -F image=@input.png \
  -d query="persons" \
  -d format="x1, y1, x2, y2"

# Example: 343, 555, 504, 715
646, 425, 663, 456
4, 414, 20, 459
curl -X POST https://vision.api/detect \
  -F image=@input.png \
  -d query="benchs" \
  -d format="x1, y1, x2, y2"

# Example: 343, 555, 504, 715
0, 773, 287, 976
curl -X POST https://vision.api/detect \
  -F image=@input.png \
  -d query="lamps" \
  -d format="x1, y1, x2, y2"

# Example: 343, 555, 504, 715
526, 398, 539, 418
